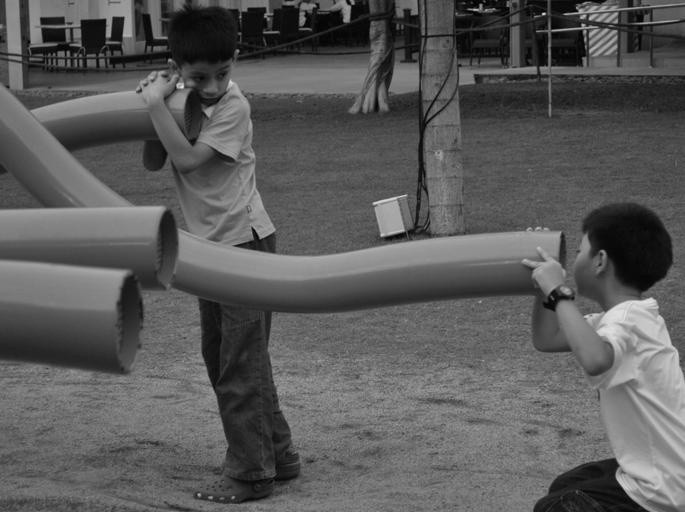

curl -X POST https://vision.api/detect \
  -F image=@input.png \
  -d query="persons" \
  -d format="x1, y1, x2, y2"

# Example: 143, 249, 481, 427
136, 2, 300, 504
521, 202, 685, 512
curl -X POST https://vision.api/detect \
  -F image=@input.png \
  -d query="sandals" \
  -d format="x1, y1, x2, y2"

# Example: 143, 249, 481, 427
275, 462, 301, 479
194, 476, 273, 503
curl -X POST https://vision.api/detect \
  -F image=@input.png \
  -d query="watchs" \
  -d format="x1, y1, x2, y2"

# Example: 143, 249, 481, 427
542, 283, 575, 310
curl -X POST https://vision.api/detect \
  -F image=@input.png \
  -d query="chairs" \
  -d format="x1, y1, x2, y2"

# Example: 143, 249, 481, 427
398, 1, 584, 72
139, 3, 350, 66
30, 11, 130, 71
